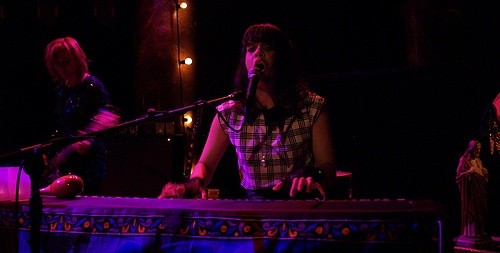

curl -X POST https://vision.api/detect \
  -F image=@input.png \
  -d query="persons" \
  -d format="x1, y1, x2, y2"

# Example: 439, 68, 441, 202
456, 139, 488, 239
156, 22, 335, 202
18, 35, 123, 195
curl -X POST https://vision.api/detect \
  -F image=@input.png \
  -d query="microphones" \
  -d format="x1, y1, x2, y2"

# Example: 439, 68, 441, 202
246, 66, 262, 103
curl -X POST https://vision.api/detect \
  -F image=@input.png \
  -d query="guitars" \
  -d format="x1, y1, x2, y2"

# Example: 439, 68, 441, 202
34, 103, 126, 186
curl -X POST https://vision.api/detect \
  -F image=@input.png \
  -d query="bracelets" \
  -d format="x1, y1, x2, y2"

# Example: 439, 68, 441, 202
315, 167, 326, 180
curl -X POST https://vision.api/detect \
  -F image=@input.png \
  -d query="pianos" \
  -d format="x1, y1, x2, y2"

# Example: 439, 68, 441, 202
21, 195, 435, 253
